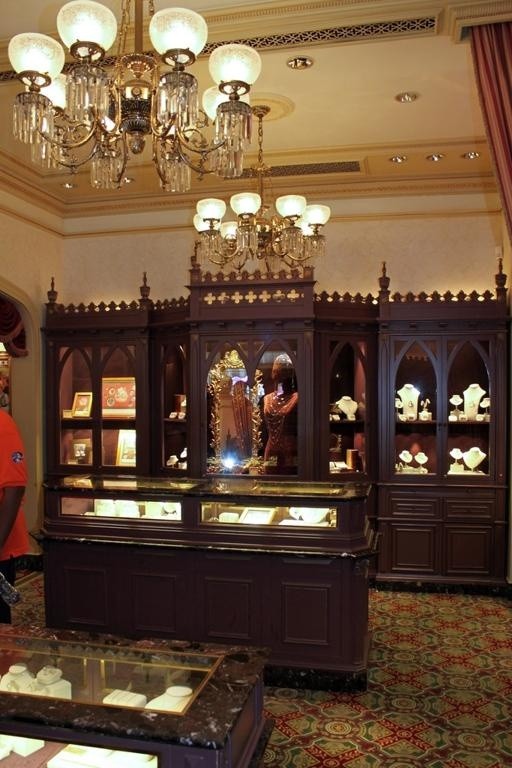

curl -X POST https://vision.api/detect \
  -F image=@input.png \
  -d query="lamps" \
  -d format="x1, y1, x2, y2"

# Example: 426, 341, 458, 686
193, 116, 331, 269
7, 0, 263, 193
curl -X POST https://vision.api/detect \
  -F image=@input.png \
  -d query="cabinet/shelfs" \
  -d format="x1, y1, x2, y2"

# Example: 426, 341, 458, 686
190, 315, 313, 482
150, 320, 192, 477
375, 317, 509, 485
313, 315, 375, 482
39, 325, 150, 492
375, 480, 510, 597
41, 481, 191, 530
191, 478, 374, 534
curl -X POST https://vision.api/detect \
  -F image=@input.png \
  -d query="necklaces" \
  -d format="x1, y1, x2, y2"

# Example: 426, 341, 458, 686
268, 393, 290, 446
7, 672, 28, 689
467, 385, 479, 409
404, 388, 413, 407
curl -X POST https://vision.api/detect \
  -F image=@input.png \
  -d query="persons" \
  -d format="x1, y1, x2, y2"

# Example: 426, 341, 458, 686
0, 410, 30, 625
